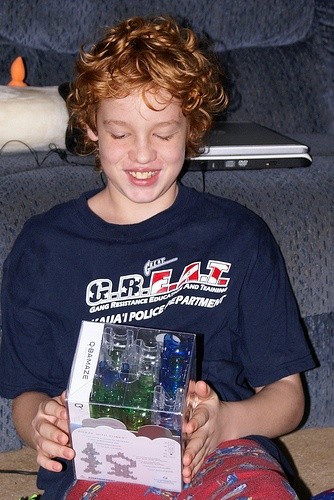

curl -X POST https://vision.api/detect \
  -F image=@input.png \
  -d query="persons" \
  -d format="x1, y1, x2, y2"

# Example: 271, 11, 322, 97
0, 15, 319, 500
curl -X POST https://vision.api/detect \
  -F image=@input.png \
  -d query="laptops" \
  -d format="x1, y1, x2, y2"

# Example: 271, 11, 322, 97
179, 121, 313, 172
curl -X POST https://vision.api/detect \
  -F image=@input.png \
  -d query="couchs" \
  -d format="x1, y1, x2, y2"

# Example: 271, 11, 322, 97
0, 0, 334, 454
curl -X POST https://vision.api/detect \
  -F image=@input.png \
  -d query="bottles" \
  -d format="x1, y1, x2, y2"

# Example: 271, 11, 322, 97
92, 331, 193, 430
